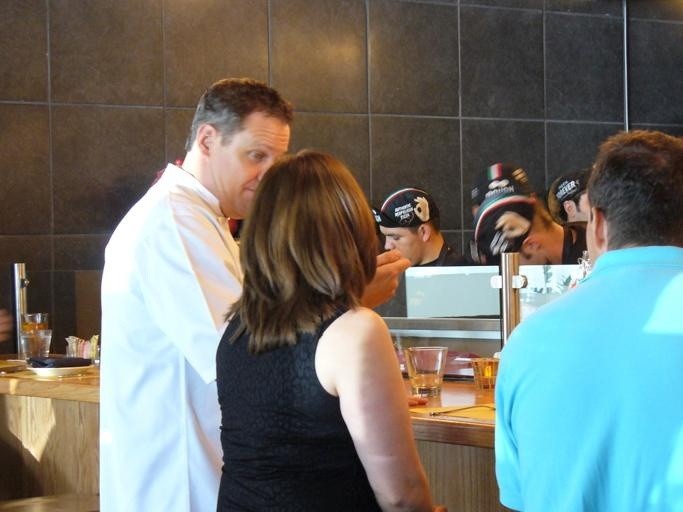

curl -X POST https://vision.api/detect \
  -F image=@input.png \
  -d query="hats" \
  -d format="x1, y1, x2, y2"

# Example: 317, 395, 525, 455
547, 173, 589, 219
371, 186, 438, 227
470, 163, 536, 266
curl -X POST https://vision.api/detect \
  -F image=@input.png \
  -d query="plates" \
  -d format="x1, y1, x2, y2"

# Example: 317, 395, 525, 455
25, 365, 95, 378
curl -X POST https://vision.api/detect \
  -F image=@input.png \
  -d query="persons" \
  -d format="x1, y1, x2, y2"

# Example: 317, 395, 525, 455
441, 163, 533, 264
551, 165, 595, 224
366, 185, 476, 267
493, 126, 682, 511
474, 190, 588, 265
213, 146, 449, 509
94, 76, 414, 512
0, 305, 14, 346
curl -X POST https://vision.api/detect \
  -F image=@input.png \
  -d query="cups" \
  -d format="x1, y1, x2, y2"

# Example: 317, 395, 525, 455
470, 357, 497, 393
20, 310, 53, 359
405, 345, 449, 396
